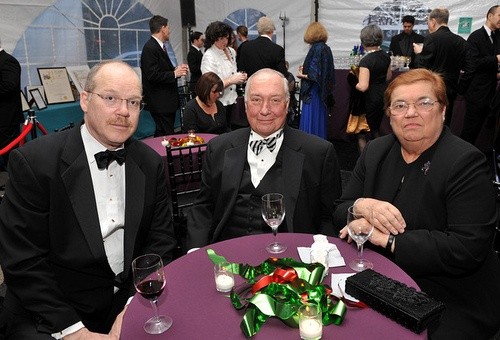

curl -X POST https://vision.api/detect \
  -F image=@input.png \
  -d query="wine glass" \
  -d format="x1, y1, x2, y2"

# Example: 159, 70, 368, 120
131, 254, 173, 334
239, 71, 245, 90
261, 193, 287, 253
347, 205, 374, 272
405, 57, 411, 67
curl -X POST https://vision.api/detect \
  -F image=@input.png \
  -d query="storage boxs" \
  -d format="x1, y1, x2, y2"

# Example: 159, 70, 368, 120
344, 267, 447, 335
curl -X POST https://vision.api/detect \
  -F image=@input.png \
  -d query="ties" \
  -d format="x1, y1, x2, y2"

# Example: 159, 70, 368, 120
163, 42, 167, 54
491, 30, 495, 42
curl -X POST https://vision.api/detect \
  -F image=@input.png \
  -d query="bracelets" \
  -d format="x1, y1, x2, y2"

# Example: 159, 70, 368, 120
303, 74, 304, 78
384, 232, 396, 255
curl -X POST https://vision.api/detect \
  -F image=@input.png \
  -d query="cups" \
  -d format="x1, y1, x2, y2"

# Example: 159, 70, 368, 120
181, 64, 188, 76
333, 56, 362, 69
310, 249, 329, 274
338, 277, 360, 309
161, 137, 169, 147
299, 303, 323, 340
214, 262, 235, 292
188, 130, 195, 138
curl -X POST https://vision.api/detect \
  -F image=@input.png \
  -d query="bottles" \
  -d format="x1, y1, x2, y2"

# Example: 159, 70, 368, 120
352, 46, 357, 56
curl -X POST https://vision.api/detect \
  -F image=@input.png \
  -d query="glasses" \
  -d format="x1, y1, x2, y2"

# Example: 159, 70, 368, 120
387, 98, 441, 111
84, 89, 146, 112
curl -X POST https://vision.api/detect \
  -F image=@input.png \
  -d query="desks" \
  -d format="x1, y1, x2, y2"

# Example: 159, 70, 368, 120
227, 83, 249, 127
329, 68, 465, 138
139, 133, 221, 185
20, 98, 156, 144
116, 230, 430, 340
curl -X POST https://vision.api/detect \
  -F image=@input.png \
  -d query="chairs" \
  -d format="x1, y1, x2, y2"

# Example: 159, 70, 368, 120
165, 142, 211, 257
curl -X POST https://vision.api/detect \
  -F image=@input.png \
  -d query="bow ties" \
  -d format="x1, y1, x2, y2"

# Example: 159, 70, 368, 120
249, 137, 276, 156
94, 149, 128, 171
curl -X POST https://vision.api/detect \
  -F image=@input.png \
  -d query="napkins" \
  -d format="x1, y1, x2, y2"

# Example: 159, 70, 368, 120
295, 233, 348, 271
330, 271, 360, 302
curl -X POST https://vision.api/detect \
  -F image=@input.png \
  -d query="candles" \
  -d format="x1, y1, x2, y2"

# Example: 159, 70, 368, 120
214, 274, 236, 292
299, 319, 322, 340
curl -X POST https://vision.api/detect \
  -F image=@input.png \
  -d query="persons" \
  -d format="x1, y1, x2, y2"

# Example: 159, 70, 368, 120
284, 61, 298, 127
185, 31, 204, 103
0, 55, 181, 340
294, 20, 335, 144
412, 8, 475, 133
336, 69, 500, 340
348, 25, 390, 159
0, 41, 25, 174
226, 30, 238, 54
388, 15, 426, 72
235, 26, 249, 55
464, 5, 500, 161
183, 68, 342, 259
138, 15, 189, 138
236, 17, 287, 129
199, 20, 247, 136
